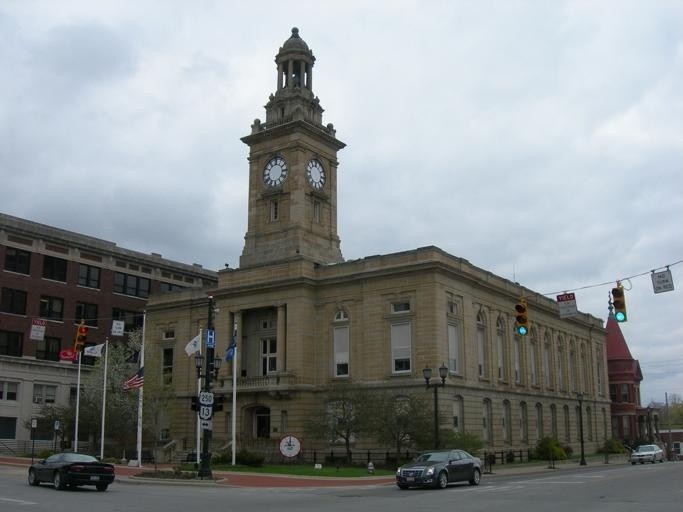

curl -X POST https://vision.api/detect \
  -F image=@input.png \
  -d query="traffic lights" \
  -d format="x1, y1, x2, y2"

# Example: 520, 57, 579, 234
190, 396, 200, 411
213, 394, 223, 412
74, 326, 88, 353
612, 286, 627, 322
513, 301, 529, 336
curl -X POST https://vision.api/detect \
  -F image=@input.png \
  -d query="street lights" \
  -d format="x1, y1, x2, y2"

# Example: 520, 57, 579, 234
422, 361, 448, 450
577, 394, 587, 466
195, 351, 222, 477
645, 406, 653, 445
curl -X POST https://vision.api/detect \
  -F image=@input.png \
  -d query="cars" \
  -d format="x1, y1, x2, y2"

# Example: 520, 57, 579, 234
396, 448, 484, 489
28, 451, 115, 491
630, 444, 665, 465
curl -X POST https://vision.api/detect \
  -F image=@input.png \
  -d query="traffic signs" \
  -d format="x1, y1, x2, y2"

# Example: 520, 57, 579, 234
29, 320, 47, 342
557, 293, 578, 320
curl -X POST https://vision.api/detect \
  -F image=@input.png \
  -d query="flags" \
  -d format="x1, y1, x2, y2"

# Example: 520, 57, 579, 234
59, 346, 78, 361
225, 334, 236, 360
184, 334, 200, 357
123, 367, 143, 389
82, 342, 105, 358
